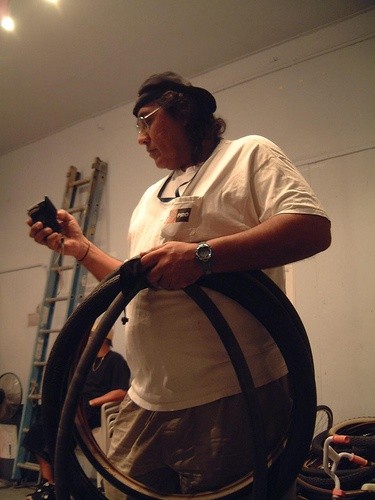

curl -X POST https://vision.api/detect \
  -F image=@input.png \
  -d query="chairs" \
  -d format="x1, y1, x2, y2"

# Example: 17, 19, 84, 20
75, 399, 122, 488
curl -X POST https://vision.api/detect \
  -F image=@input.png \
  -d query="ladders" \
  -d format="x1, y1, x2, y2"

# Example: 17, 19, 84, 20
11, 157, 108, 491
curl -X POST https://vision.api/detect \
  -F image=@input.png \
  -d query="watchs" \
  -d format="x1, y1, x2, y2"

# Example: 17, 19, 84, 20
193, 241, 215, 277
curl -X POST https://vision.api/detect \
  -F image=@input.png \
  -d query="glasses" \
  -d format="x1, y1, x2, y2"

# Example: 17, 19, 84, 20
136, 106, 163, 133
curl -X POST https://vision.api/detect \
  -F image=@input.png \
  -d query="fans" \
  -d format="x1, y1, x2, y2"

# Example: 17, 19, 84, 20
0, 372, 22, 424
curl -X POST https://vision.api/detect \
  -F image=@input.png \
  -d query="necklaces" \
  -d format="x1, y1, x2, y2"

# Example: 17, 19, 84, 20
157, 159, 204, 203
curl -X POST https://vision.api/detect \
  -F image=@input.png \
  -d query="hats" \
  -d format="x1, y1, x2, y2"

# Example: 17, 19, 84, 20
90, 317, 113, 340
133, 71, 217, 118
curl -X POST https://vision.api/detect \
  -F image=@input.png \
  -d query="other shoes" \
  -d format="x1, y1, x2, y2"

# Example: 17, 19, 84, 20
25, 480, 56, 500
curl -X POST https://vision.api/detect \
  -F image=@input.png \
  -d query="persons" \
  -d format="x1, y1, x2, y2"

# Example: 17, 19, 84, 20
26, 321, 131, 500
24, 70, 331, 500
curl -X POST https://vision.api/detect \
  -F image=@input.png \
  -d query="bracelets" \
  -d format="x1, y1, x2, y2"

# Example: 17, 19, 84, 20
78, 240, 91, 262
